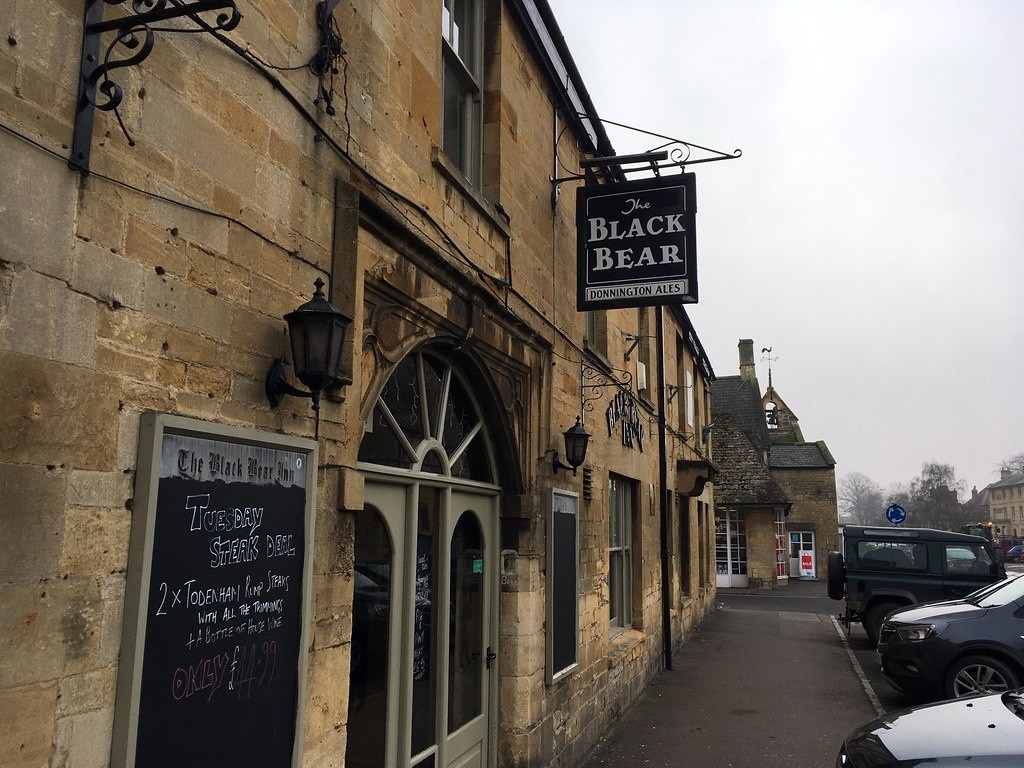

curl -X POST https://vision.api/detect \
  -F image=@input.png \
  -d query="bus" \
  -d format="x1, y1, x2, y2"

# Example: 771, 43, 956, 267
960, 523, 994, 554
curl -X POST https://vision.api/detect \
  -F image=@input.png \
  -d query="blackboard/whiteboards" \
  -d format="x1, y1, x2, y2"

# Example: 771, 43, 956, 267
414, 552, 433, 682
112, 415, 321, 768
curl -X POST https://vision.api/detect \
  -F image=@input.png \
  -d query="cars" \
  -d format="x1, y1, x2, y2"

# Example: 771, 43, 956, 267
835, 686, 1024, 768
1006, 544, 1024, 563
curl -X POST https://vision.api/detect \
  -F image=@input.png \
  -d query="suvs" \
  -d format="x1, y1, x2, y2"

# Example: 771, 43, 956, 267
877, 572, 1024, 704
827, 525, 1009, 645
